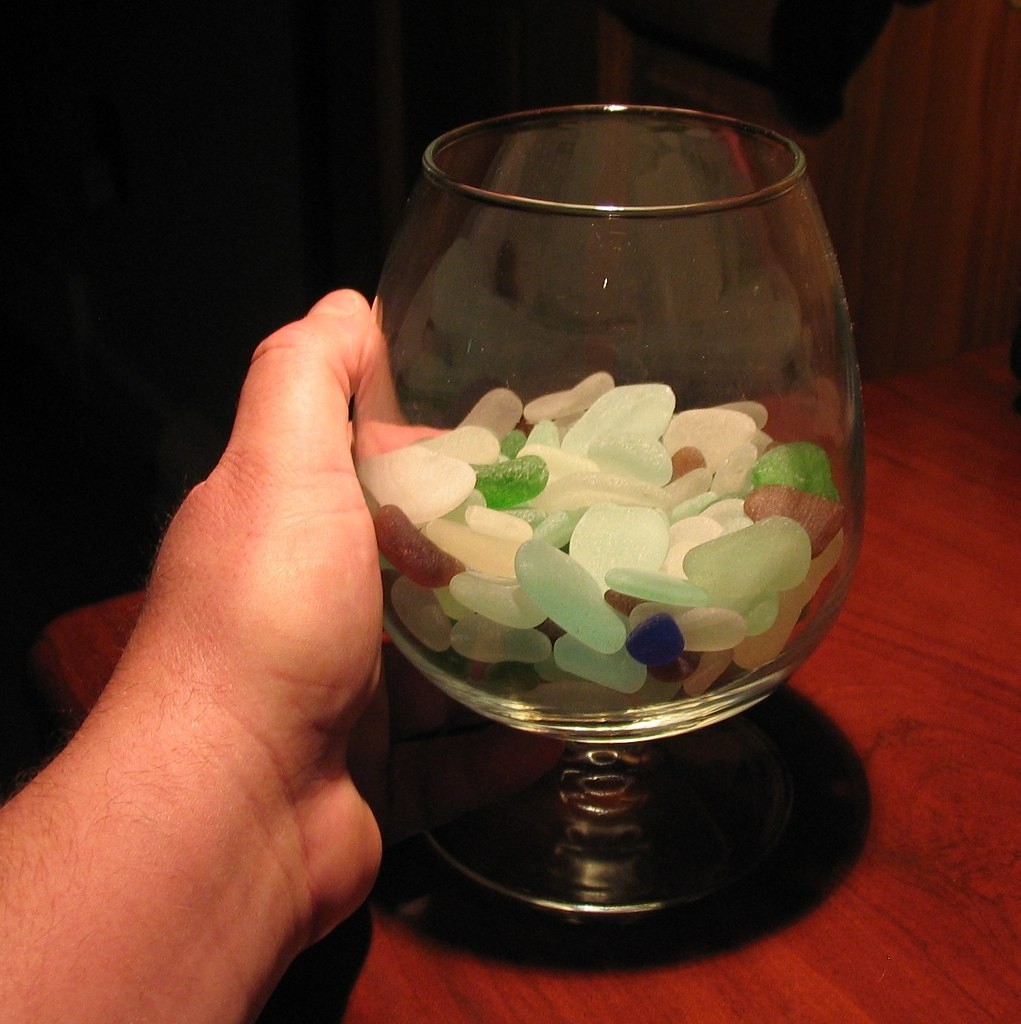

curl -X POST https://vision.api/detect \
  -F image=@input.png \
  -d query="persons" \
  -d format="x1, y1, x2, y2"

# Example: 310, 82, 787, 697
1, 0, 894, 802
0, 289, 382, 1024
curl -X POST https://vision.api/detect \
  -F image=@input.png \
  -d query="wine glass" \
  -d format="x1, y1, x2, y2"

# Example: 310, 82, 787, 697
352, 107, 863, 918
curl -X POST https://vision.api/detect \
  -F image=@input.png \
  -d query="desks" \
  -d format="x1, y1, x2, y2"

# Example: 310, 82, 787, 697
61, 344, 1021, 1024
